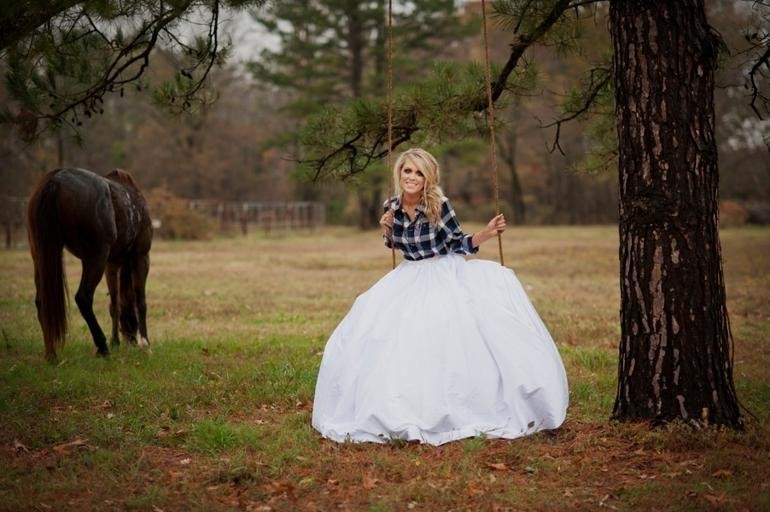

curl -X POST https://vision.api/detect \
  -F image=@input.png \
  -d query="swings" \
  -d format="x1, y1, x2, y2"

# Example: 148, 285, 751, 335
384, 2, 508, 271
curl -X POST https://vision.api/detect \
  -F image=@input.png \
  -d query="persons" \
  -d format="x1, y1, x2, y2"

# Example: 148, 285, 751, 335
311, 148, 570, 447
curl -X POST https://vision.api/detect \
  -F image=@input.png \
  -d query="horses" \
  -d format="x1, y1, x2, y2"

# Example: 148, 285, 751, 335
24, 164, 152, 364
